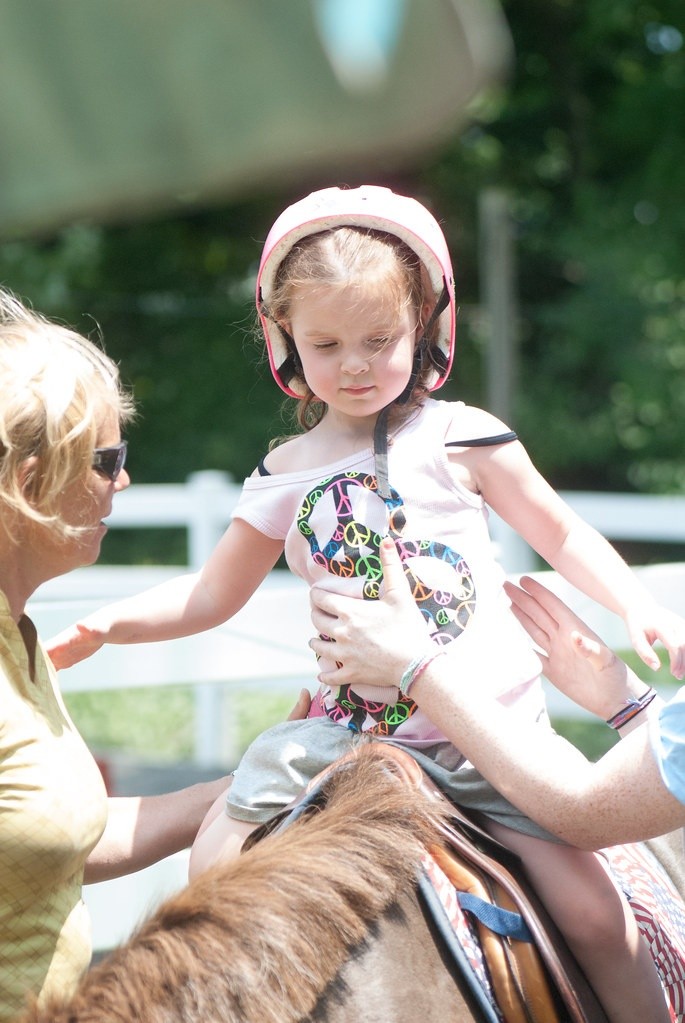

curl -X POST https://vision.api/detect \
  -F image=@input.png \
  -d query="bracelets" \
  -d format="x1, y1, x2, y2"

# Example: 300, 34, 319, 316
397, 645, 447, 697
605, 686, 657, 730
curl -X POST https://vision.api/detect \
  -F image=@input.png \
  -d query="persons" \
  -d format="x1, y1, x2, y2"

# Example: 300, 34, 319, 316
0, 185, 685, 1023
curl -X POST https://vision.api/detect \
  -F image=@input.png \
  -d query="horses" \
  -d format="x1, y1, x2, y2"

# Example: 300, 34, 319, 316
12, 734, 685, 1023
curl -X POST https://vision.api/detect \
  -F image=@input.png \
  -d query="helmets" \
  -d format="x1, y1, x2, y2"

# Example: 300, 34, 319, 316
255, 185, 456, 402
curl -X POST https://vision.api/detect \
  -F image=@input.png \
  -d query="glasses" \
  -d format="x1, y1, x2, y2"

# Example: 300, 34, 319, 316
0, 439, 128, 484
